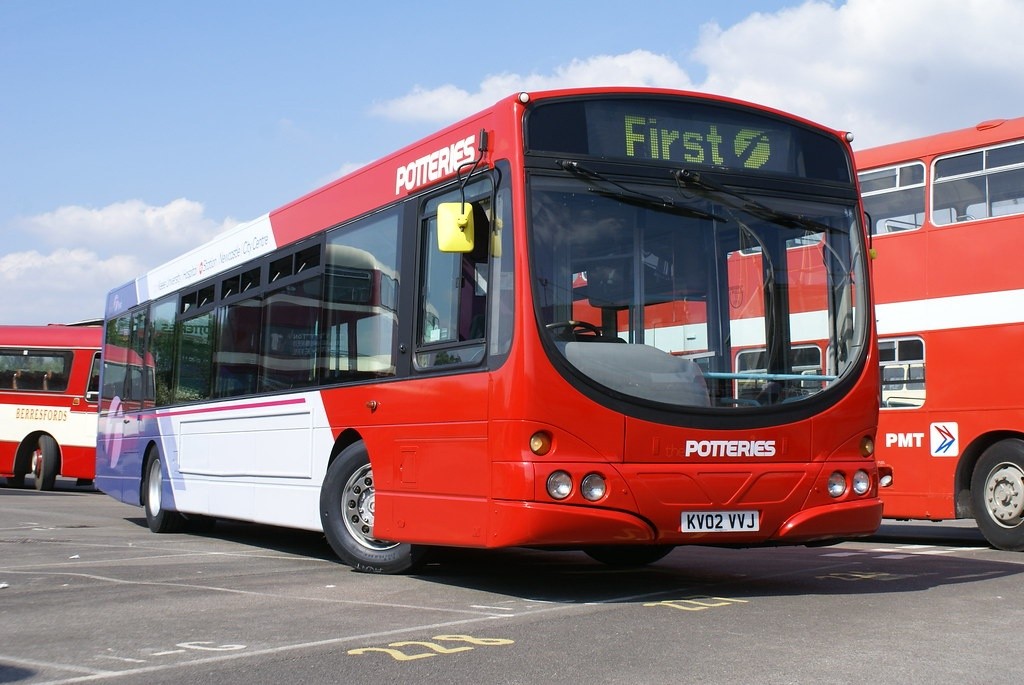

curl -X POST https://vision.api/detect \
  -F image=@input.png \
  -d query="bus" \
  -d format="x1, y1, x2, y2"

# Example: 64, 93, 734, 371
101, 342, 157, 415
94, 86, 892, 574
0, 324, 103, 492
572, 115, 1024, 551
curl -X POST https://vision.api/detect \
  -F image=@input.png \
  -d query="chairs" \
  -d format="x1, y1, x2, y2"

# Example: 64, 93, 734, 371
0, 369, 67, 391
